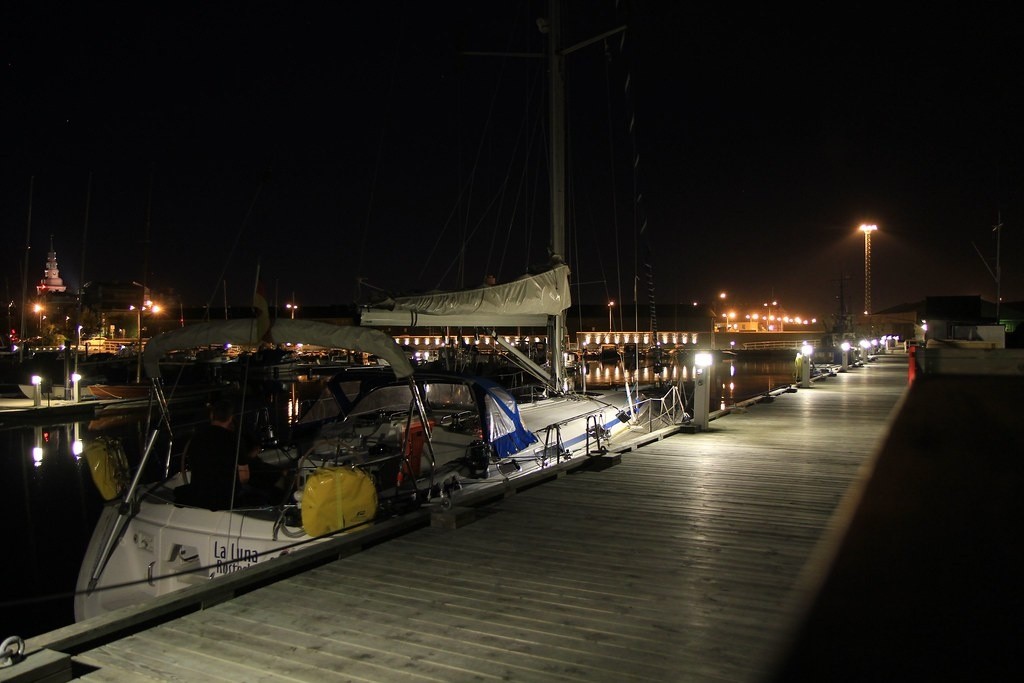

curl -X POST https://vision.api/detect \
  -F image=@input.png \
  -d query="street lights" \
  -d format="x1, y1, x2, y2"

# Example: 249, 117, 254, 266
35, 305, 41, 331
861, 224, 878, 316
609, 302, 613, 332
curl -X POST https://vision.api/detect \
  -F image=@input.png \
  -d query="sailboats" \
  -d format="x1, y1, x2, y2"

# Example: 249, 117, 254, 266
68, 0, 657, 626
644, 318, 662, 360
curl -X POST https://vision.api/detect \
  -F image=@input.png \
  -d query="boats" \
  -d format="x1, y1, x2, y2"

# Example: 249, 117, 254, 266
599, 343, 620, 365
16, 374, 163, 400
621, 342, 641, 359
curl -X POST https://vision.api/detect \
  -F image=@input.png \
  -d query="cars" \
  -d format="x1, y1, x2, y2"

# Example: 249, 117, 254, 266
82, 337, 107, 346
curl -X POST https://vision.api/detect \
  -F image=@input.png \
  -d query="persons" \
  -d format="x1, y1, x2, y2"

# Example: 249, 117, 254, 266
192, 400, 261, 509
